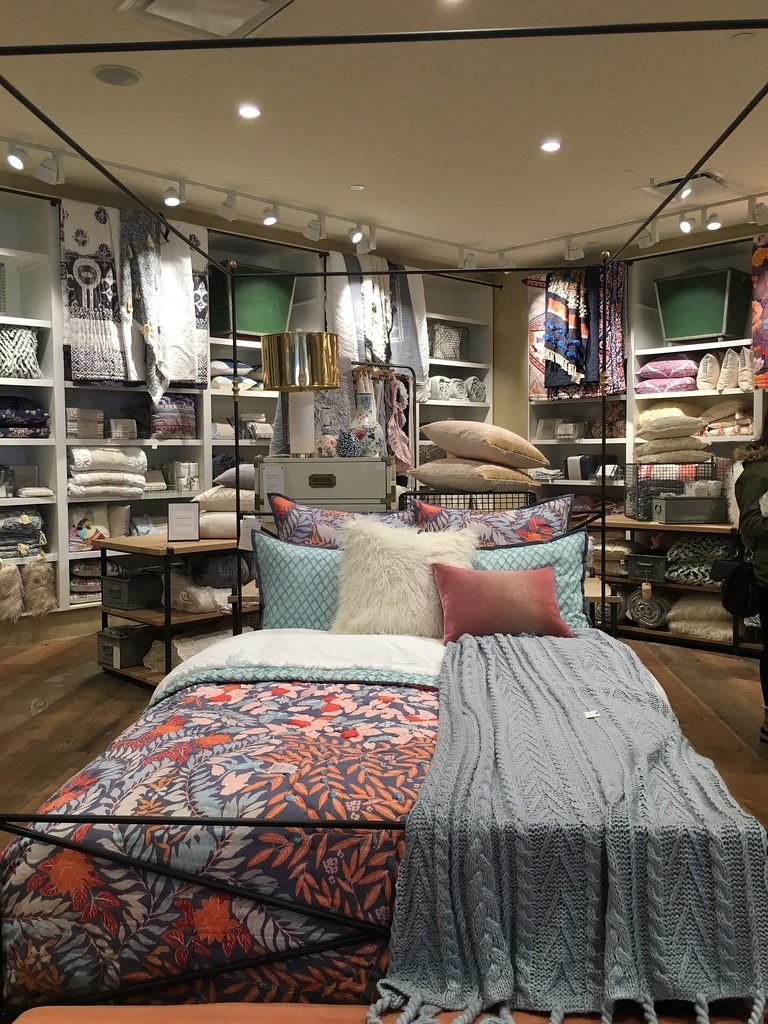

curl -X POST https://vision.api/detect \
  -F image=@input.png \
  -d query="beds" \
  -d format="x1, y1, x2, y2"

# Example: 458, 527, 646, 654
1, 531, 767, 1004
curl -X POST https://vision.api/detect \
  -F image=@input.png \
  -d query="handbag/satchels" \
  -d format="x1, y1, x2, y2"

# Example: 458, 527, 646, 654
721, 563, 759, 618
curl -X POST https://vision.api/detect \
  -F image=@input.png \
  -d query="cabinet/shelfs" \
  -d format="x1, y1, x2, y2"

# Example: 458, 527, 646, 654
0, 184, 494, 619
528, 231, 768, 530
89, 535, 261, 685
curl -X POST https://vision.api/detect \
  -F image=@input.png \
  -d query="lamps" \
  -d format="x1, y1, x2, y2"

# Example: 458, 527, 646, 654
0, 145, 768, 272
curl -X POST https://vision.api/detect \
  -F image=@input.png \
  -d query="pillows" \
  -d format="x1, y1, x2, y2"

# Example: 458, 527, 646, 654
589, 539, 650, 576
213, 464, 255, 490
432, 563, 576, 646
406, 457, 542, 492
191, 511, 256, 539
189, 486, 256, 514
266, 492, 418, 550
419, 421, 551, 468
411, 493, 575, 547
634, 344, 753, 464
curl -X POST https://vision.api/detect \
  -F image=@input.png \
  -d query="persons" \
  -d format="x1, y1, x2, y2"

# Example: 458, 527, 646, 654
735, 408, 768, 744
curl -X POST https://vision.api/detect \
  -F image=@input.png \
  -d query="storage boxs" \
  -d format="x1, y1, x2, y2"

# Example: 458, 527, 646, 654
96, 623, 154, 670
101, 575, 162, 611
650, 496, 729, 524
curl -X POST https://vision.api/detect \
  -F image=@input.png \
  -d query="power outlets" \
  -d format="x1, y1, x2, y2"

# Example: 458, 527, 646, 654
586, 515, 768, 652
